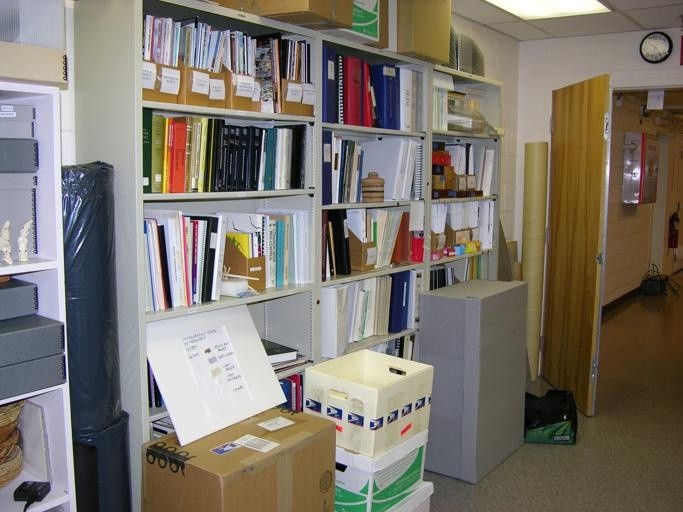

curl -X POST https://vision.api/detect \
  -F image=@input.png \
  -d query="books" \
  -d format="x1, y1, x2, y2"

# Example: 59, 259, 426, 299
142, 14, 311, 114
322, 201, 425, 283
433, 70, 493, 135
322, 45, 423, 132
431, 200, 494, 261
142, 108, 314, 194
322, 130, 426, 206
430, 254, 490, 291
320, 267, 426, 361
261, 338, 311, 412
432, 137, 494, 200
143, 208, 309, 311
147, 357, 176, 440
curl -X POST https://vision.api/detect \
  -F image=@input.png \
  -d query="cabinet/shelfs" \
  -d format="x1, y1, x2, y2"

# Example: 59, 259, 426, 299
0, 82, 84, 512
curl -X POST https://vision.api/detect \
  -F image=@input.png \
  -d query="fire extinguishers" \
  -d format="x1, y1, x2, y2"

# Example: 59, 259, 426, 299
668, 212, 679, 248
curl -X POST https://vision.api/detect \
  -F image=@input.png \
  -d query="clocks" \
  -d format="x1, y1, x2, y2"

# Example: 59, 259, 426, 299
640, 31, 673, 63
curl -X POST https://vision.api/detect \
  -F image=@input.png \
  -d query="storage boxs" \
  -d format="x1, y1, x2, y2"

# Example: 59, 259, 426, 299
420, 279, 528, 484
